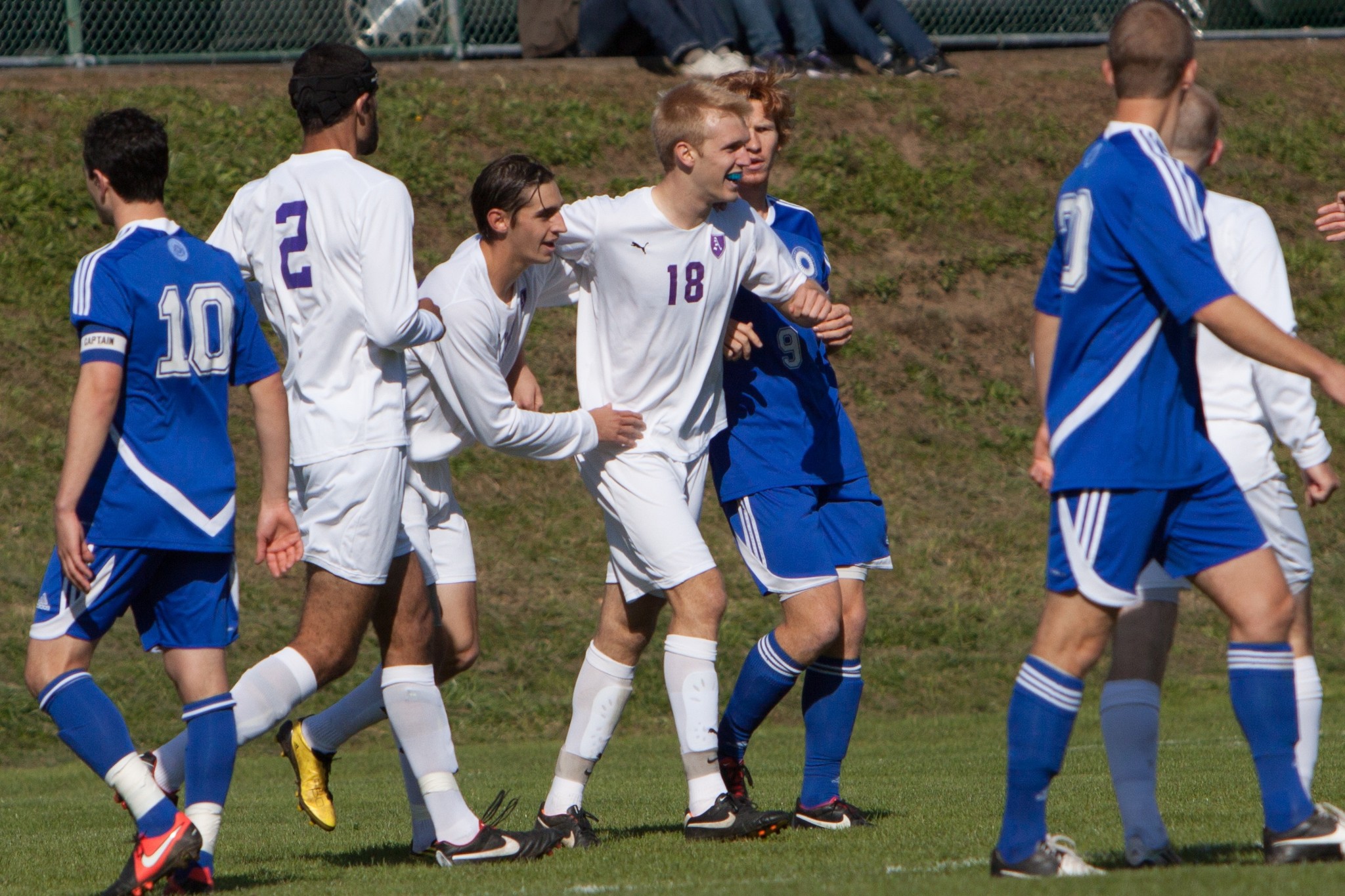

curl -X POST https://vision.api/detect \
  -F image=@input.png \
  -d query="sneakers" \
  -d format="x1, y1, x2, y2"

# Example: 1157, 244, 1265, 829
1262, 802, 1345, 865
98, 811, 203, 896
795, 794, 874, 832
990, 832, 1109, 882
717, 51, 751, 77
717, 752, 755, 806
275, 712, 336, 832
684, 793, 791, 843
163, 857, 214, 896
877, 54, 922, 79
535, 801, 602, 850
746, 52, 800, 79
916, 52, 959, 76
670, 44, 730, 81
795, 50, 852, 79
406, 788, 510, 865
435, 796, 564, 871
113, 751, 178, 818
1120, 846, 1183, 869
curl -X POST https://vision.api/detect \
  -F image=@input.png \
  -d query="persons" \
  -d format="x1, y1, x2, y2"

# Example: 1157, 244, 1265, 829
24, 108, 305, 896
276, 156, 646, 830
1098, 84, 1339, 869
116, 40, 563, 865
1315, 190, 1345, 242
505, 79, 832, 846
709, 70, 894, 833
990, 0, 1345, 879
518, 0, 960, 78
